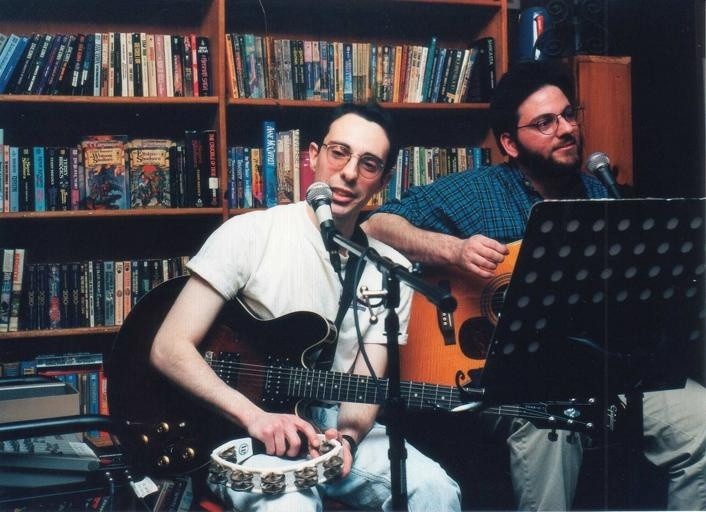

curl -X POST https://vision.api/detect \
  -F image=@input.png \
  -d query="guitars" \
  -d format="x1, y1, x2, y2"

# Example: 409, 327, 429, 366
385, 237, 524, 388
106, 273, 597, 476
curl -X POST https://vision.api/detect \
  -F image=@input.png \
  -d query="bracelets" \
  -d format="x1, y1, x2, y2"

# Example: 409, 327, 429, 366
341, 432, 358, 457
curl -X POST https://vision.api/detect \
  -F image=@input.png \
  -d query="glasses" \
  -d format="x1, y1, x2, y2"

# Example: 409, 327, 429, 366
321, 143, 384, 179
517, 107, 585, 135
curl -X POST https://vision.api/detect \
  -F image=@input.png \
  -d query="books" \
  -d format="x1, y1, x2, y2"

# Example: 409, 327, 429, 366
0, 120, 492, 214
2, 246, 195, 510
0, 30, 494, 105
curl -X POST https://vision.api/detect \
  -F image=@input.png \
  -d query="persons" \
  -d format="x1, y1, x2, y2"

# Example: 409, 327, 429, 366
147, 100, 465, 511
363, 63, 706, 512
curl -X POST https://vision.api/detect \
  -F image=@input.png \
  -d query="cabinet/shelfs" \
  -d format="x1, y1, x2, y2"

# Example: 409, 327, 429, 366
0, 0, 511, 450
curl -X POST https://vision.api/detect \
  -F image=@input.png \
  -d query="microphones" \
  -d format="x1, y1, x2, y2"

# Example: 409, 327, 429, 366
586, 152, 622, 198
305, 181, 345, 271
